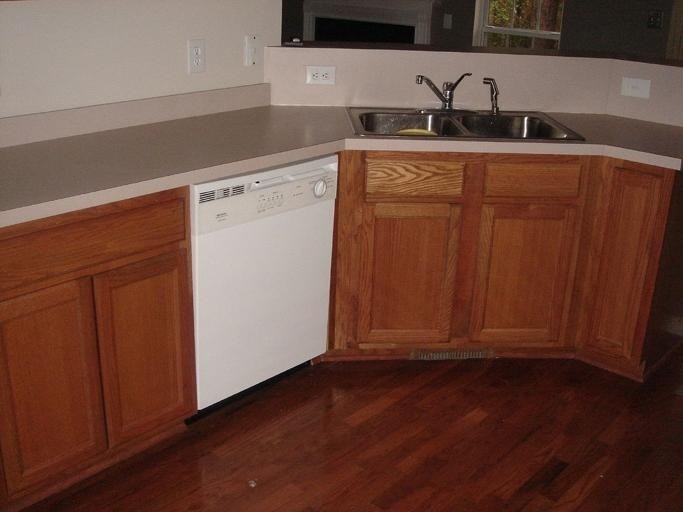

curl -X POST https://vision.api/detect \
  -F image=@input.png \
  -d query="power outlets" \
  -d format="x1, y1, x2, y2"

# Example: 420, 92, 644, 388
306, 65, 337, 85
187, 38, 205, 74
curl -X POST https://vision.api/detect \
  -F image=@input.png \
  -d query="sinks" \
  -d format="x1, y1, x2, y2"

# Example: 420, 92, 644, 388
450, 110, 586, 142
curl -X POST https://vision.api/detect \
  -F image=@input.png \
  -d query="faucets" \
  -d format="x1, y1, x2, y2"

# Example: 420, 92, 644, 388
415, 73, 472, 109
481, 76, 500, 113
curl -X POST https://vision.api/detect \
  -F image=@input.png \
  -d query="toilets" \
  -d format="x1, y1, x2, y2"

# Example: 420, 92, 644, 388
343, 106, 470, 137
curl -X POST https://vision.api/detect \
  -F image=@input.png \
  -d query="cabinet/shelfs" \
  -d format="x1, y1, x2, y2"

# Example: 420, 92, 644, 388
326, 150, 683, 383
1, 184, 197, 512
